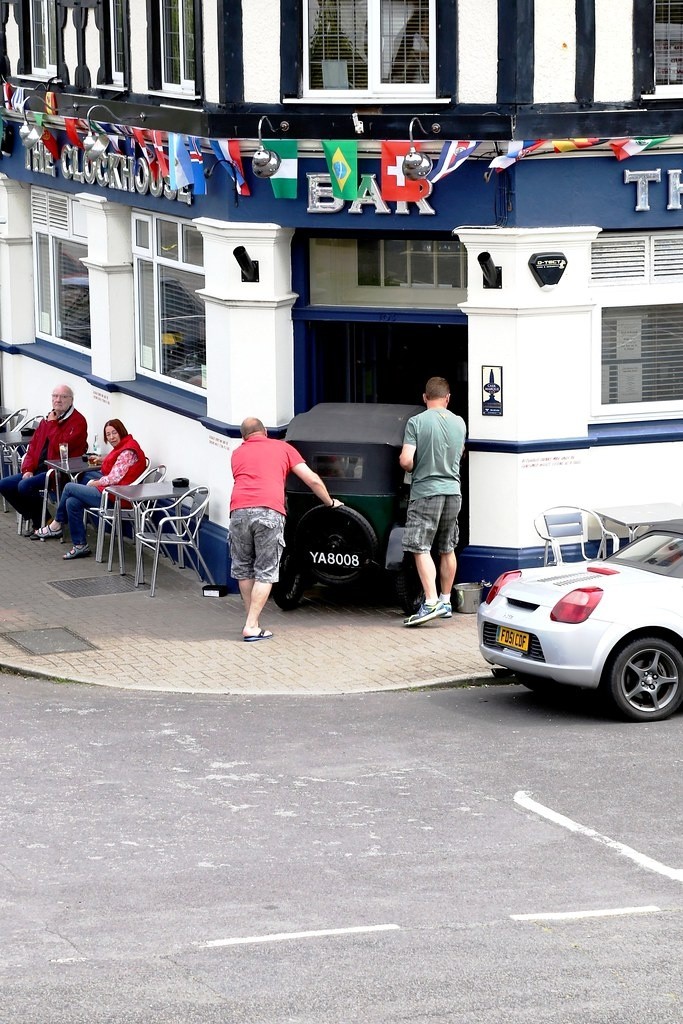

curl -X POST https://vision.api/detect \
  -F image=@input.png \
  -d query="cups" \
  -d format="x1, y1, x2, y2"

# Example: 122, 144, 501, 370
82, 455, 89, 463
59, 443, 68, 460
88, 456, 97, 466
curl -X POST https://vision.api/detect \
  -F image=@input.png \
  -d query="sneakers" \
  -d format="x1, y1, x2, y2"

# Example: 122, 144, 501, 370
403, 600, 448, 626
437, 601, 452, 618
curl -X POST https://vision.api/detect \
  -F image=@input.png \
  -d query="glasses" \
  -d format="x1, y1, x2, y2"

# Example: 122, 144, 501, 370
52, 394, 73, 399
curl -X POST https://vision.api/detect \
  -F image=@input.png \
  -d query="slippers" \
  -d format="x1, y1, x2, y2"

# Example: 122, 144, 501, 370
243, 629, 273, 641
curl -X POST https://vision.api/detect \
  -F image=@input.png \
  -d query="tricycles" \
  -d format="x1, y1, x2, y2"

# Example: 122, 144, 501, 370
271, 401, 429, 618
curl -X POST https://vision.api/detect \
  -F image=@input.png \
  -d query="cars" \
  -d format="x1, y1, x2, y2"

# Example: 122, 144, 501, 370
476, 524, 682, 723
58, 251, 206, 387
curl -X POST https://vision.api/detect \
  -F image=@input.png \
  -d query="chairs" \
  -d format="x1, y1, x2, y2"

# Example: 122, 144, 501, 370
0, 408, 216, 597
533, 506, 620, 567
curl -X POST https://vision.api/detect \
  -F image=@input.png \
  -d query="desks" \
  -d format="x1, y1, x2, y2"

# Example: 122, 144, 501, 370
105, 481, 198, 585
43, 456, 102, 543
0, 433, 33, 513
593, 503, 683, 559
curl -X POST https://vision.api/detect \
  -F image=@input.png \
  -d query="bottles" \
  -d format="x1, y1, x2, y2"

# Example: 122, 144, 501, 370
94, 436, 100, 455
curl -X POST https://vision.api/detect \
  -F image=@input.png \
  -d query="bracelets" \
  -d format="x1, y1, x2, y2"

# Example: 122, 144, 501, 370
328, 501, 334, 507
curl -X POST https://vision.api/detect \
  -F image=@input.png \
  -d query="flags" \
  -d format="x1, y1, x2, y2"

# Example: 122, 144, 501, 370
381, 141, 421, 202
209, 140, 251, 195
34, 112, 207, 195
44, 92, 56, 115
489, 137, 671, 172
427, 141, 482, 184
262, 140, 298, 198
3, 83, 24, 110
322, 141, 358, 200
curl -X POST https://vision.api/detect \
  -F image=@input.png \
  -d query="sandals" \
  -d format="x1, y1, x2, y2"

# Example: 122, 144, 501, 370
34, 525, 63, 539
63, 543, 92, 560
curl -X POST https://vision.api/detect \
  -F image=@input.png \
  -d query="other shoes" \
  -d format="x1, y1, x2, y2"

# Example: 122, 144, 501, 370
29, 534, 41, 541
24, 529, 34, 537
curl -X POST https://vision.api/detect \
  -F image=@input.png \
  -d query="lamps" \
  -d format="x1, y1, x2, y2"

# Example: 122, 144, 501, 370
20, 95, 68, 149
83, 104, 145, 161
402, 117, 441, 182
252, 116, 290, 178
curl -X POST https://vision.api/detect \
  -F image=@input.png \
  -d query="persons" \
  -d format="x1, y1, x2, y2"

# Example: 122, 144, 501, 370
35, 419, 147, 560
0, 385, 88, 542
398, 376, 466, 628
230, 418, 344, 641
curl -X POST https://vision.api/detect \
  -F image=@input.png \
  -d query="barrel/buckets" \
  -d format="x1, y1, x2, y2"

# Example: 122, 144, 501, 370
454, 583, 483, 613
